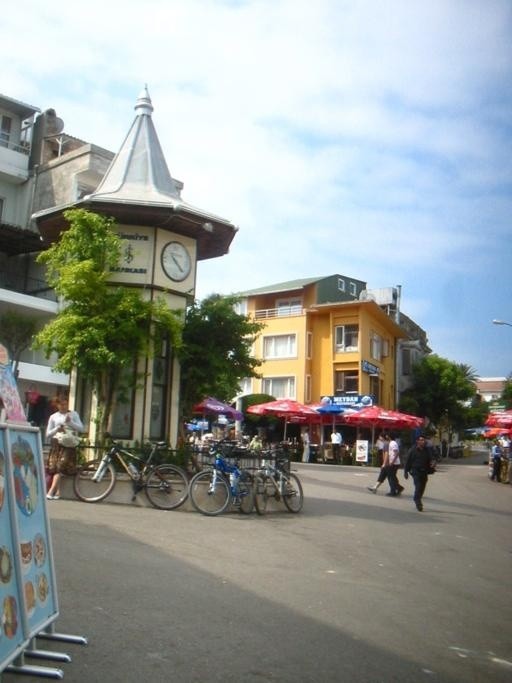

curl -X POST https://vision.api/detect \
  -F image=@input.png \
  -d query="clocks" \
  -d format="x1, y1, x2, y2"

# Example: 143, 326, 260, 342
160, 241, 192, 282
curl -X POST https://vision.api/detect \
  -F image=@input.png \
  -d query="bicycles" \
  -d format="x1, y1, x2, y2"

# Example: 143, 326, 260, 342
253, 447, 303, 514
188, 435, 255, 516
74, 429, 190, 510
497, 456, 511, 482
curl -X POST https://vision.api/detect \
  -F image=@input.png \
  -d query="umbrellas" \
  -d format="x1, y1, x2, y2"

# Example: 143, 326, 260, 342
247, 397, 426, 446
481, 409, 512, 439
192, 395, 245, 441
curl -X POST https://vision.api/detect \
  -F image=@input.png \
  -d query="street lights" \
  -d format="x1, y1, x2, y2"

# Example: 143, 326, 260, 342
493, 320, 512, 327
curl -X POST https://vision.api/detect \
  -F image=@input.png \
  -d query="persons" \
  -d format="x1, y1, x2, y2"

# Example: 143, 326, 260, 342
189, 431, 199, 446
300, 426, 311, 462
47, 394, 84, 501
366, 432, 403, 496
491, 440, 512, 484
331, 429, 342, 465
404, 436, 434, 511
249, 436, 262, 449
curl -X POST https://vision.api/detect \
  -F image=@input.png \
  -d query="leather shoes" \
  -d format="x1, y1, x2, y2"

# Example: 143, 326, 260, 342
397, 486, 404, 494
47, 494, 53, 500
368, 486, 377, 493
54, 494, 62, 500
386, 492, 394, 497
417, 506, 422, 511
414, 499, 422, 506
